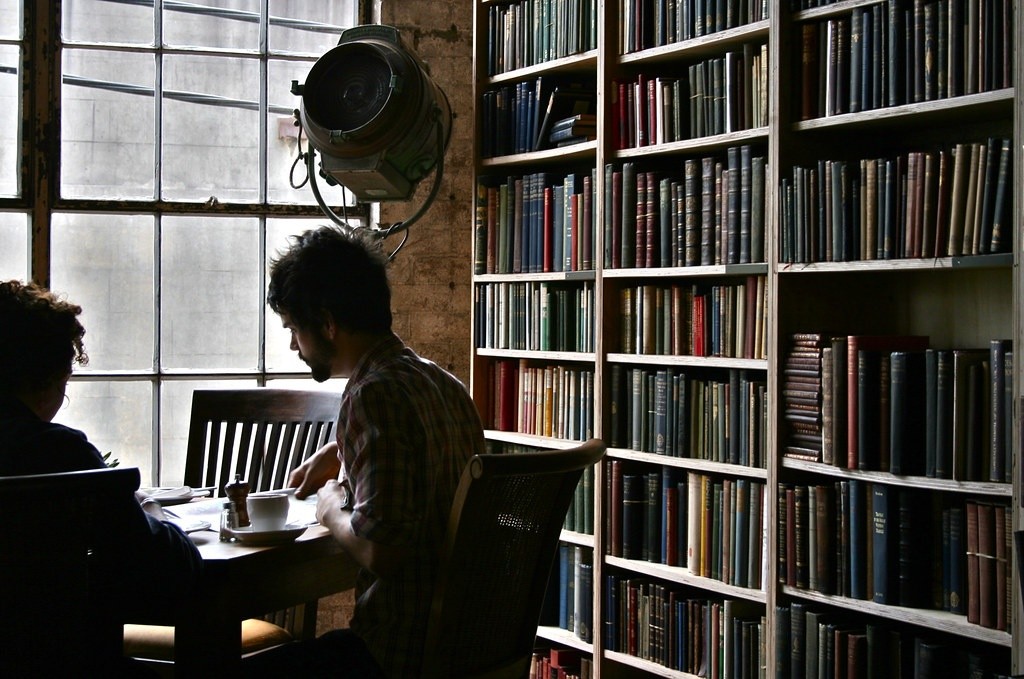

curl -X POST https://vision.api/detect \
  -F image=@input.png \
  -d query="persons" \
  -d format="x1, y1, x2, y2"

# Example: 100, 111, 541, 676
0, 280, 201, 679
259, 226, 526, 679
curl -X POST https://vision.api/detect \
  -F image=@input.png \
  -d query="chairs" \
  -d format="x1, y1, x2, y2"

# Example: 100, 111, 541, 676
122, 390, 341, 679
0, 465, 140, 679
417, 438, 606, 679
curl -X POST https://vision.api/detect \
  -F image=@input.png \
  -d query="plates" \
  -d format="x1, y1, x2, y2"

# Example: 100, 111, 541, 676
167, 518, 210, 535
231, 524, 308, 546
150, 487, 210, 506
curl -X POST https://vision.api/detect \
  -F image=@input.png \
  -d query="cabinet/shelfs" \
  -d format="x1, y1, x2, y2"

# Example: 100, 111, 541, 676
471, 0, 1024, 679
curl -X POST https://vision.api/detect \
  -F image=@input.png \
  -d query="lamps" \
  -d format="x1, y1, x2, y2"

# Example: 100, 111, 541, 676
291, 21, 452, 263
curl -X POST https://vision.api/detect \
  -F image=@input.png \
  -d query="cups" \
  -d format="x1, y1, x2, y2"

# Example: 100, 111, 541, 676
246, 494, 289, 532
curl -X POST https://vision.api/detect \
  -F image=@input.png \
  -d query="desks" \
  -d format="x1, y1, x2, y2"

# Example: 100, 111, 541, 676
118, 498, 363, 679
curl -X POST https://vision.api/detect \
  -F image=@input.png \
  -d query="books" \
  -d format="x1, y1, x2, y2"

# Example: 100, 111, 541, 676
474, 0, 1023, 678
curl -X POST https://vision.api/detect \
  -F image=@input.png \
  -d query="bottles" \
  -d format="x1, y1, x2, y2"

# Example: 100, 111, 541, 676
219, 501, 239, 542
